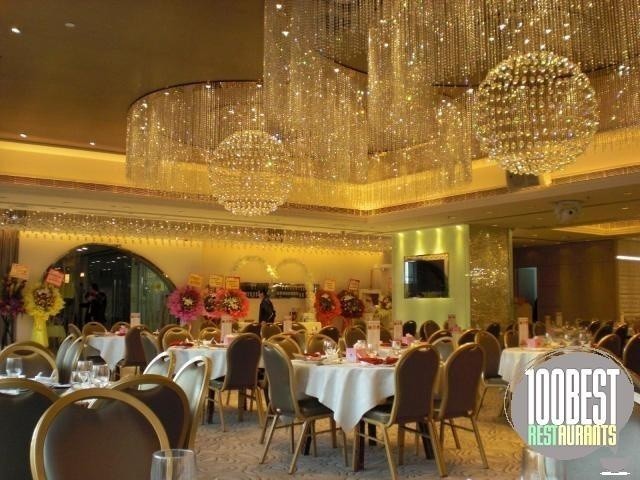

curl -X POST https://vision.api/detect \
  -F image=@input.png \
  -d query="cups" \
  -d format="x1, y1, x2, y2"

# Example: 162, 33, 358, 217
6, 358, 24, 377
70, 359, 110, 389
150, 450, 200, 480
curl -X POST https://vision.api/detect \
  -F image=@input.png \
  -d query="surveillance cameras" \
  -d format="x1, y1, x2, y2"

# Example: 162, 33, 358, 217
563, 209, 577, 216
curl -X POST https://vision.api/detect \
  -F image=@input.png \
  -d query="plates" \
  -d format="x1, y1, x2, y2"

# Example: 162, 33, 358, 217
47, 381, 70, 390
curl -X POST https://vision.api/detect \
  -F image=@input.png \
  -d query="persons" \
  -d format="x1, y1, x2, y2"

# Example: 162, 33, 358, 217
257, 287, 275, 323
84, 283, 108, 325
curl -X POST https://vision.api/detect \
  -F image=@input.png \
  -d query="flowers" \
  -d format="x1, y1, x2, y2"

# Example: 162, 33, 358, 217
22, 282, 66, 323
166, 285, 366, 320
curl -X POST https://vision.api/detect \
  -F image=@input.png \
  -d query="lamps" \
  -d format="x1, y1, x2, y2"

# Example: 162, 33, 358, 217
124, 72, 469, 224
258, 0, 640, 191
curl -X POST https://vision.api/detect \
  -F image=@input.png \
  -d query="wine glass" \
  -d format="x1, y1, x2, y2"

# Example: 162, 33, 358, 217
530, 319, 596, 347
315, 337, 400, 365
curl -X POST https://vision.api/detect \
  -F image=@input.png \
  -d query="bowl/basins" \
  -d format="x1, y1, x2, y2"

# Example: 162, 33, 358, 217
29, 375, 58, 383
185, 341, 225, 348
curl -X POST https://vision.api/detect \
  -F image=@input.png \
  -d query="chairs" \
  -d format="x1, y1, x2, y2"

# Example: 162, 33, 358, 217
2, 309, 639, 480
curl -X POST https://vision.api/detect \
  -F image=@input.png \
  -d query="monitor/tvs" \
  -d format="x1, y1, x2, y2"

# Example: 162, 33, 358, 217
403, 254, 450, 299
